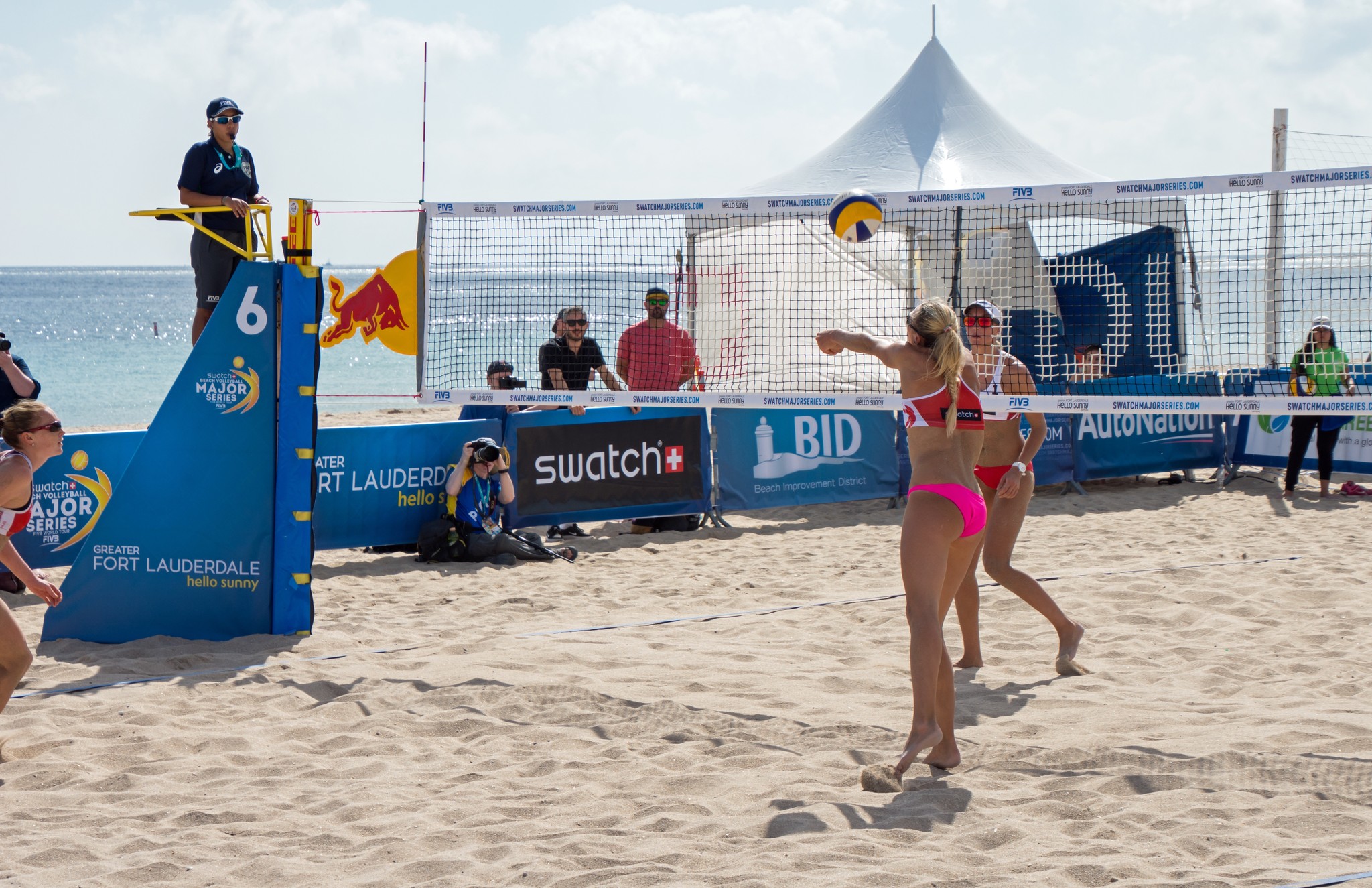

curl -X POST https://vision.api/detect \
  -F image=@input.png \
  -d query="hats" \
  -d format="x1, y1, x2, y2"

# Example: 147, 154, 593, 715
206, 97, 244, 116
487, 360, 513, 375
1312, 316, 1333, 330
963, 299, 1002, 325
646, 287, 670, 300
552, 308, 567, 333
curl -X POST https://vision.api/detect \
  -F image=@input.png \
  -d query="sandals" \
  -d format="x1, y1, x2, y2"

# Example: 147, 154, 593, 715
559, 545, 578, 561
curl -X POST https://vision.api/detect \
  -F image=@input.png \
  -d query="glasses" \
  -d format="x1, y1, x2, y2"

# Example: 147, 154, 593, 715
566, 319, 587, 327
964, 316, 997, 328
16, 420, 62, 433
649, 299, 668, 306
207, 115, 241, 124
491, 364, 514, 371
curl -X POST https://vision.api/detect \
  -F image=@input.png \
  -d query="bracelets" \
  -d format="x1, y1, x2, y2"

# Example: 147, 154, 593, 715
498, 468, 509, 474
221, 196, 230, 206
255, 195, 270, 204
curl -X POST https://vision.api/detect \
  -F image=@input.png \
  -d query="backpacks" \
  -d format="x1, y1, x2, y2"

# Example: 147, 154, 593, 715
416, 518, 482, 563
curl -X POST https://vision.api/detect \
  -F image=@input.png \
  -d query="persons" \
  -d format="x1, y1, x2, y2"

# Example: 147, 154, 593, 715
0, 332, 50, 579
816, 300, 988, 776
951, 301, 1084, 669
177, 97, 272, 348
445, 437, 579, 560
458, 359, 560, 444
617, 288, 696, 393
0, 397, 67, 713
538, 307, 641, 541
1066, 344, 1115, 395
1276, 317, 1357, 500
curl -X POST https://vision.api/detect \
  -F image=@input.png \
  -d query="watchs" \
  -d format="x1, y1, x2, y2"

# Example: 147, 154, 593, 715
1011, 462, 1027, 476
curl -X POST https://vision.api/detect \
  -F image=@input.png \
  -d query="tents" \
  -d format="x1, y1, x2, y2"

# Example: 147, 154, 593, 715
685, 4, 1185, 395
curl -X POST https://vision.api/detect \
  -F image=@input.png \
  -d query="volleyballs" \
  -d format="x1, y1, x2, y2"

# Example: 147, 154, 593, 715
1289, 375, 1317, 396
828, 188, 883, 242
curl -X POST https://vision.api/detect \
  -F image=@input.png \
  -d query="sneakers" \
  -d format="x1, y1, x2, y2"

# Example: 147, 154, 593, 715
545, 524, 564, 543
1341, 480, 1372, 498
562, 524, 593, 540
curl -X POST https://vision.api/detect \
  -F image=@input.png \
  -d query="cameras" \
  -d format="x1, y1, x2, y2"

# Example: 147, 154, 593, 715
468, 438, 500, 464
498, 376, 527, 392
